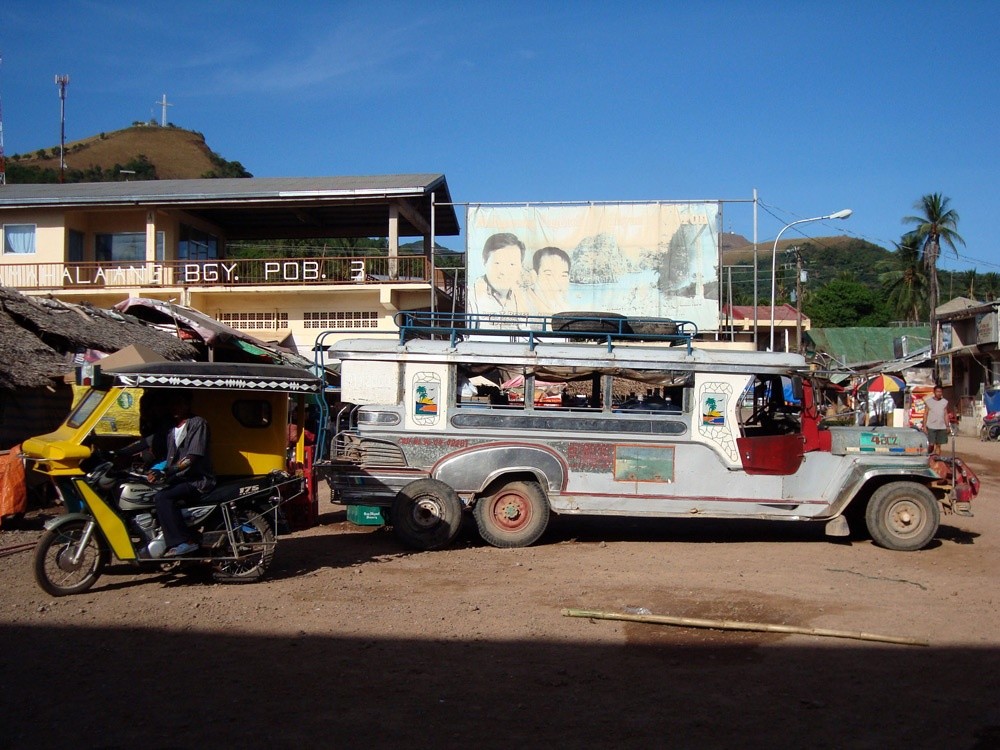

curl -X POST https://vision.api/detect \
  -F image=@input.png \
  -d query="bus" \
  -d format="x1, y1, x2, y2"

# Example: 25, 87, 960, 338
316, 311, 984, 554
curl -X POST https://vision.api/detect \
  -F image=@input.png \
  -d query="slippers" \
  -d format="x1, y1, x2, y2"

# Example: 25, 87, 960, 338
165, 545, 199, 557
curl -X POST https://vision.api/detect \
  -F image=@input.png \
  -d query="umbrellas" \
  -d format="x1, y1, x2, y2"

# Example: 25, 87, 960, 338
857, 373, 906, 410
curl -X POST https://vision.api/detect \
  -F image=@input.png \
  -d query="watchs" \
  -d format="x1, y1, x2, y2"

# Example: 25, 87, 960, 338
160, 470, 166, 477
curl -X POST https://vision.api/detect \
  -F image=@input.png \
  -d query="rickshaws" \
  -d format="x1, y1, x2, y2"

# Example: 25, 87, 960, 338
15, 361, 329, 598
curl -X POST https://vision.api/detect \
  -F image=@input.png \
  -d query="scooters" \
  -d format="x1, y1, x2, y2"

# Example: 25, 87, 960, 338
982, 412, 1000, 442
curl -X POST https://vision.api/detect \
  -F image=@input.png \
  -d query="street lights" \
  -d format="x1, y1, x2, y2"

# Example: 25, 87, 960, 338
770, 205, 853, 352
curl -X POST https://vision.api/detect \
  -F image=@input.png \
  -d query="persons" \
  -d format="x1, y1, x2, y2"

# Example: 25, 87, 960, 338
103, 394, 210, 558
922, 386, 951, 455
466, 232, 571, 330
